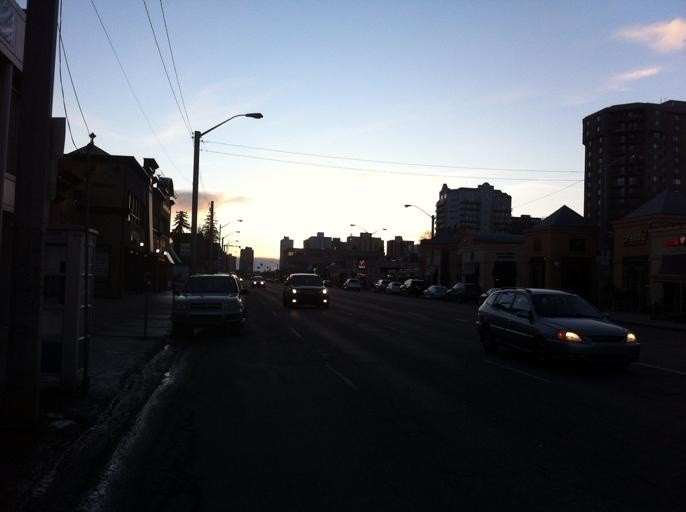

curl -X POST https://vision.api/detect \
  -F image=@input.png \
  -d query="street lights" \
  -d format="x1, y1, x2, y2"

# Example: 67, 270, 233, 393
191, 113, 264, 272
403, 203, 435, 289
349, 223, 386, 238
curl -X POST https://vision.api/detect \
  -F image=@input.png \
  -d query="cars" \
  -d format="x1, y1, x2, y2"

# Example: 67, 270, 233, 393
174, 273, 249, 336
375, 279, 482, 303
478, 289, 640, 370
344, 279, 361, 291
323, 279, 333, 287
282, 273, 331, 309
252, 275, 268, 288
